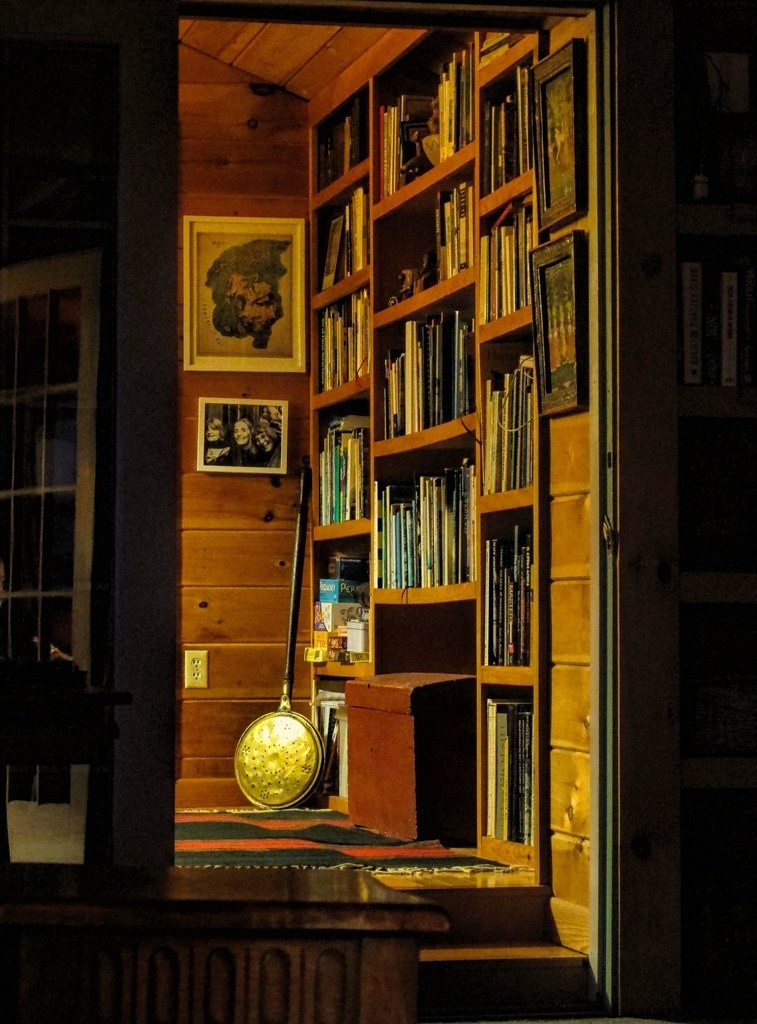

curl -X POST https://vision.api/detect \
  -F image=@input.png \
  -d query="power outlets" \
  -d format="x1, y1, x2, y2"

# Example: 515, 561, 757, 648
185, 650, 208, 689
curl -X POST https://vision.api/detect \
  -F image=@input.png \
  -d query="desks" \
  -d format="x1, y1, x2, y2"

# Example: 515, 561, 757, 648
0, 863, 453, 1024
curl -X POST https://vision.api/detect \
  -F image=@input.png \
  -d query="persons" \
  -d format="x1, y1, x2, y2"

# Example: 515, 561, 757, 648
205, 406, 282, 469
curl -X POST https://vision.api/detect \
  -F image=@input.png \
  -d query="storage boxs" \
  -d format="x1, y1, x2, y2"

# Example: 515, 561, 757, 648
313, 630, 337, 647
347, 622, 369, 653
303, 647, 345, 662
319, 579, 358, 603
320, 602, 362, 632
349, 652, 369, 663
329, 557, 369, 579
344, 672, 476, 841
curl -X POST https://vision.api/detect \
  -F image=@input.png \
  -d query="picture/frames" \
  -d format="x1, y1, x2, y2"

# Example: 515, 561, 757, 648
196, 397, 289, 475
529, 229, 588, 420
531, 37, 589, 236
182, 215, 307, 374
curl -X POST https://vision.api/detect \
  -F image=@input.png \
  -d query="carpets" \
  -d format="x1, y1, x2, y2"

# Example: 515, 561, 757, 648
176, 807, 536, 875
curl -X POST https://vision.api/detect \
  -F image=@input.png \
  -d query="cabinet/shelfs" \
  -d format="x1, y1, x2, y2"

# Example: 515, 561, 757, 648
614, 0, 757, 1024
309, 28, 544, 872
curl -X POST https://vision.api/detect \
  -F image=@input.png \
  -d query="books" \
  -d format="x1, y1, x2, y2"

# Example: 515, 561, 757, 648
385, 311, 474, 439
484, 523, 531, 667
479, 207, 534, 322
484, 356, 535, 496
314, 90, 371, 193
314, 185, 371, 392
482, 60, 536, 199
478, 698, 534, 846
308, 694, 344, 799
377, 39, 477, 201
317, 416, 368, 527
426, 182, 476, 284
375, 457, 474, 590
